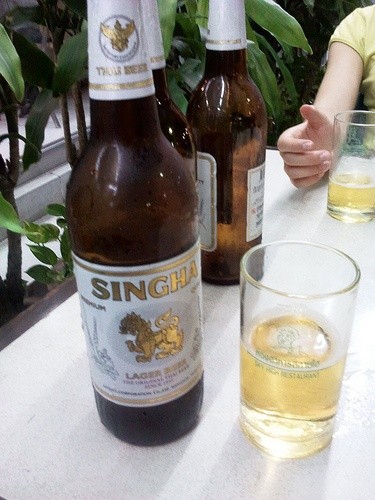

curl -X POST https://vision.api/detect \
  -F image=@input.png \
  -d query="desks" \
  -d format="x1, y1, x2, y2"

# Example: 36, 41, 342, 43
0, 147, 375, 499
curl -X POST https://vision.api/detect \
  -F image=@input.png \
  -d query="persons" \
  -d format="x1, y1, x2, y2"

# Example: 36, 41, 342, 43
277, 4, 375, 188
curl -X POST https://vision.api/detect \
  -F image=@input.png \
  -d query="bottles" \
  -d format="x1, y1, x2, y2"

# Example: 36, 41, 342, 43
67, 0, 203, 447
184, 0, 268, 287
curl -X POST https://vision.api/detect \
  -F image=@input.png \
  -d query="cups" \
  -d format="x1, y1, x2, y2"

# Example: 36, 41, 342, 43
325, 110, 375, 224
236, 240, 360, 462
